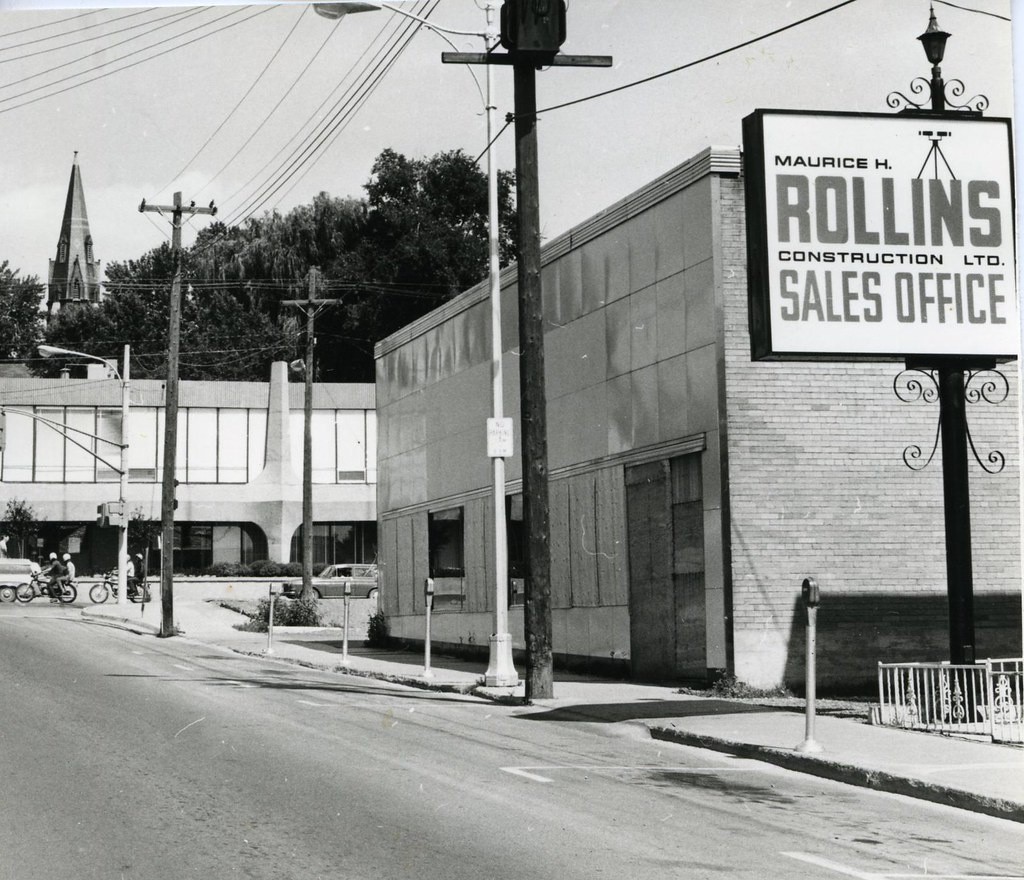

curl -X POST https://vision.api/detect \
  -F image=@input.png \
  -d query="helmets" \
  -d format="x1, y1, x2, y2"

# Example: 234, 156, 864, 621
49, 552, 58, 561
61, 553, 71, 562
126, 554, 130, 561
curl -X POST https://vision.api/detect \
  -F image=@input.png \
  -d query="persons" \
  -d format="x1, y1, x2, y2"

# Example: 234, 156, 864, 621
112, 553, 146, 596
0, 534, 9, 558
43, 552, 75, 603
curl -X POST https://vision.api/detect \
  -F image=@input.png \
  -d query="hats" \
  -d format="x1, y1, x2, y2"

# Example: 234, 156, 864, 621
136, 553, 143, 560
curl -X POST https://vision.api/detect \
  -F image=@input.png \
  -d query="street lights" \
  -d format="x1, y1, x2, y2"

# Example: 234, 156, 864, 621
36, 345, 129, 604
313, 3, 527, 702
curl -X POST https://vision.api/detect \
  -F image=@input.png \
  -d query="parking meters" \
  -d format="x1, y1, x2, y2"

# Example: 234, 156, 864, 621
420, 577, 438, 679
339, 580, 354, 666
794, 576, 826, 755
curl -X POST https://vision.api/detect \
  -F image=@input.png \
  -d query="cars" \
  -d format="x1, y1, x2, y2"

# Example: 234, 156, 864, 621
282, 563, 378, 601
0, 557, 52, 603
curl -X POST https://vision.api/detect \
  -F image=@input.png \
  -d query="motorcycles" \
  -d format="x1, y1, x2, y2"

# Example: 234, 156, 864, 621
15, 570, 79, 604
89, 565, 152, 604
